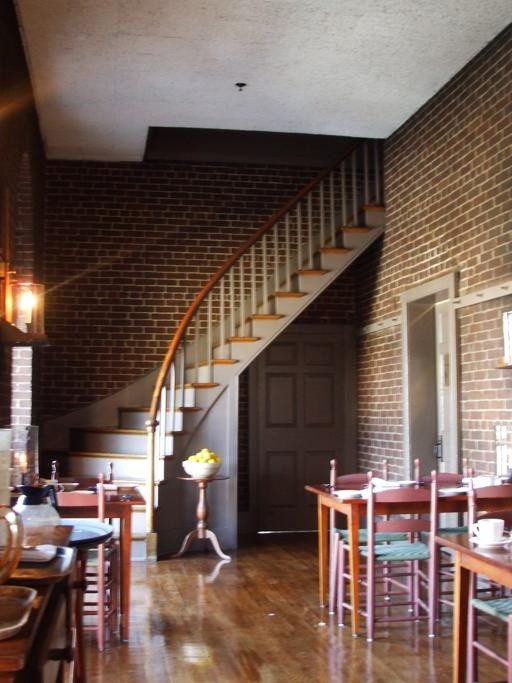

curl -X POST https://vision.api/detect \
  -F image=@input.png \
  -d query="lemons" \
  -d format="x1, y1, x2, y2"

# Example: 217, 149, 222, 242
186, 448, 221, 464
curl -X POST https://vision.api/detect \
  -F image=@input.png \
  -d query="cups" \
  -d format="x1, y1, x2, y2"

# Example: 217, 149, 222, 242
471, 518, 507, 541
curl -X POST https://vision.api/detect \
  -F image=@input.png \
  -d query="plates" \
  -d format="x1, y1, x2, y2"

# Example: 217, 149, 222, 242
333, 489, 362, 499
110, 482, 137, 490
466, 537, 511, 549
439, 487, 467, 495
398, 480, 420, 487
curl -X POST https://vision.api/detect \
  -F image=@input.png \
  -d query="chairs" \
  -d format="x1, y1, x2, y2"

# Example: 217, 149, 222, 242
407, 456, 471, 611
327, 457, 412, 616
338, 468, 437, 642
51, 459, 123, 484
464, 468, 512, 533
48, 473, 117, 649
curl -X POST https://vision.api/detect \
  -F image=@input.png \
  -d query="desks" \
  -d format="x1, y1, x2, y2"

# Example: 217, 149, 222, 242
305, 478, 512, 638
170, 472, 232, 561
47, 485, 147, 643
434, 533, 512, 682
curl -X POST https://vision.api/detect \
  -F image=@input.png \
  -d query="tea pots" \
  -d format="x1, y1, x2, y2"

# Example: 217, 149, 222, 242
9, 483, 60, 555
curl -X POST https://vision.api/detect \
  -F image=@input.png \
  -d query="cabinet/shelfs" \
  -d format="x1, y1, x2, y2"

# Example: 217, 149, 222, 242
0, 541, 86, 682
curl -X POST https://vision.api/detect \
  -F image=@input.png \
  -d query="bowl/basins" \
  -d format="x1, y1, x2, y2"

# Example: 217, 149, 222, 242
59, 482, 80, 490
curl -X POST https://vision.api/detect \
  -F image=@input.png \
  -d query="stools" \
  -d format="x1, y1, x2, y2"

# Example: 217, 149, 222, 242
467, 588, 512, 682
